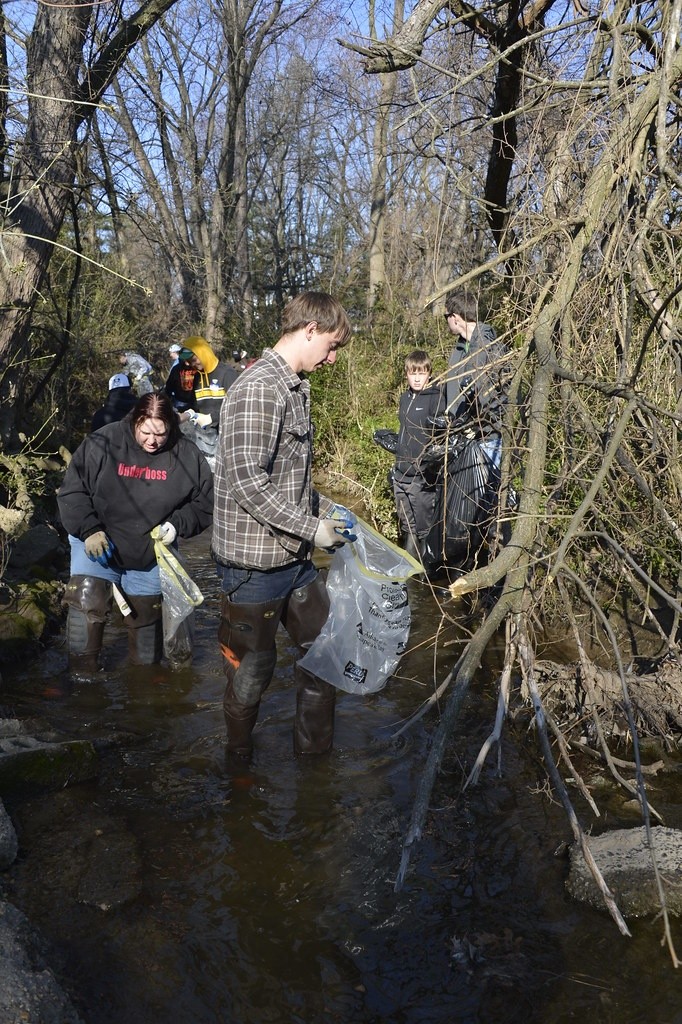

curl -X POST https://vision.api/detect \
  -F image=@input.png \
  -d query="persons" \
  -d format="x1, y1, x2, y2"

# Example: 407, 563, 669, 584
164, 335, 240, 436
168, 344, 183, 375
166, 348, 201, 404
56, 392, 214, 683
228, 348, 249, 373
91, 373, 141, 432
208, 290, 359, 762
116, 351, 158, 398
443, 289, 512, 584
393, 350, 446, 564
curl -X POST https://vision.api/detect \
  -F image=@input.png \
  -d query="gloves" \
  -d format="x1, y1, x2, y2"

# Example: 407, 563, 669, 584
135, 375, 142, 381
188, 413, 212, 429
326, 504, 357, 524
84, 530, 114, 569
158, 521, 177, 546
308, 518, 357, 555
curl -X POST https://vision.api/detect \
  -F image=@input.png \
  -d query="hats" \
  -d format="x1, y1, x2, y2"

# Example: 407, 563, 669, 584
178, 348, 195, 360
168, 344, 182, 353
108, 373, 132, 391
232, 348, 243, 359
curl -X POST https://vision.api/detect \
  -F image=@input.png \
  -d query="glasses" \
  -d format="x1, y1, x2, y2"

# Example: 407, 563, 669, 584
444, 312, 455, 320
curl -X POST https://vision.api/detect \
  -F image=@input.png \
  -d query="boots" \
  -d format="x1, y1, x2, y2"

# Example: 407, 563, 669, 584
64, 575, 113, 673
120, 595, 164, 667
277, 572, 337, 755
216, 593, 286, 755
